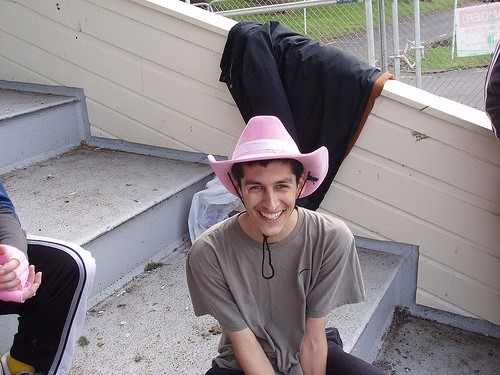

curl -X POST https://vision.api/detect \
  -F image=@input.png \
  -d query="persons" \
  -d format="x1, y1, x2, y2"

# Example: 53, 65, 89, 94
186, 116, 387, 375
0, 181, 96, 375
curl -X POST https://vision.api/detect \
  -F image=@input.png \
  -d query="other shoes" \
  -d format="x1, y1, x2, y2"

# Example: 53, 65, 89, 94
1, 351, 37, 375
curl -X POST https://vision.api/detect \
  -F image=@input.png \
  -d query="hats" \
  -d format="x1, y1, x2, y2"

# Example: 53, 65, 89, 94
208, 115, 330, 200
